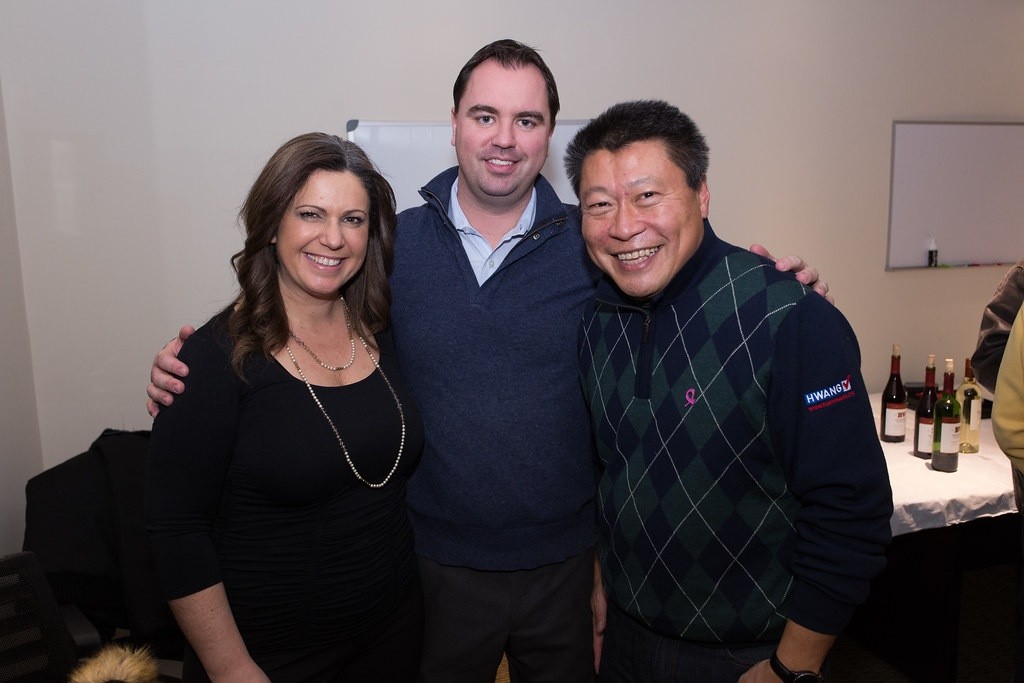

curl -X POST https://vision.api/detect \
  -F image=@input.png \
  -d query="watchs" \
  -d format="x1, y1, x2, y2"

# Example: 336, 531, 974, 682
770, 650, 824, 683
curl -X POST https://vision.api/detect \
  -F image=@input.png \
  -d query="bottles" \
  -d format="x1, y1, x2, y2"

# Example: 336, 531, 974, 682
957, 358, 982, 453
880, 344, 908, 443
914, 355, 937, 459
931, 359, 961, 472
928, 239, 938, 267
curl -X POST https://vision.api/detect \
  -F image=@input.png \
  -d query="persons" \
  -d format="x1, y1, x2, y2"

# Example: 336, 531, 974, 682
563, 100, 893, 683
970, 258, 1024, 515
147, 40, 837, 683
144, 133, 424, 683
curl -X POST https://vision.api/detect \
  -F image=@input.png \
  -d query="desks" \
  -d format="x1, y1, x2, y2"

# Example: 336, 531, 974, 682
868, 392, 1014, 527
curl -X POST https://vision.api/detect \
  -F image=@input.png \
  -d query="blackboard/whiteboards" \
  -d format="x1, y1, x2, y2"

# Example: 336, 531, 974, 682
345, 120, 596, 214
885, 121, 1024, 272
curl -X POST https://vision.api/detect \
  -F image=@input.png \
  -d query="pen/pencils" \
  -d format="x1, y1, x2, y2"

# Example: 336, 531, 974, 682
937, 264, 966, 267
968, 263, 1002, 267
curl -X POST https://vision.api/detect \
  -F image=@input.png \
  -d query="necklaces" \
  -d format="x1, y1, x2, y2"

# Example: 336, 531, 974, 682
283, 294, 405, 487
288, 303, 356, 370
